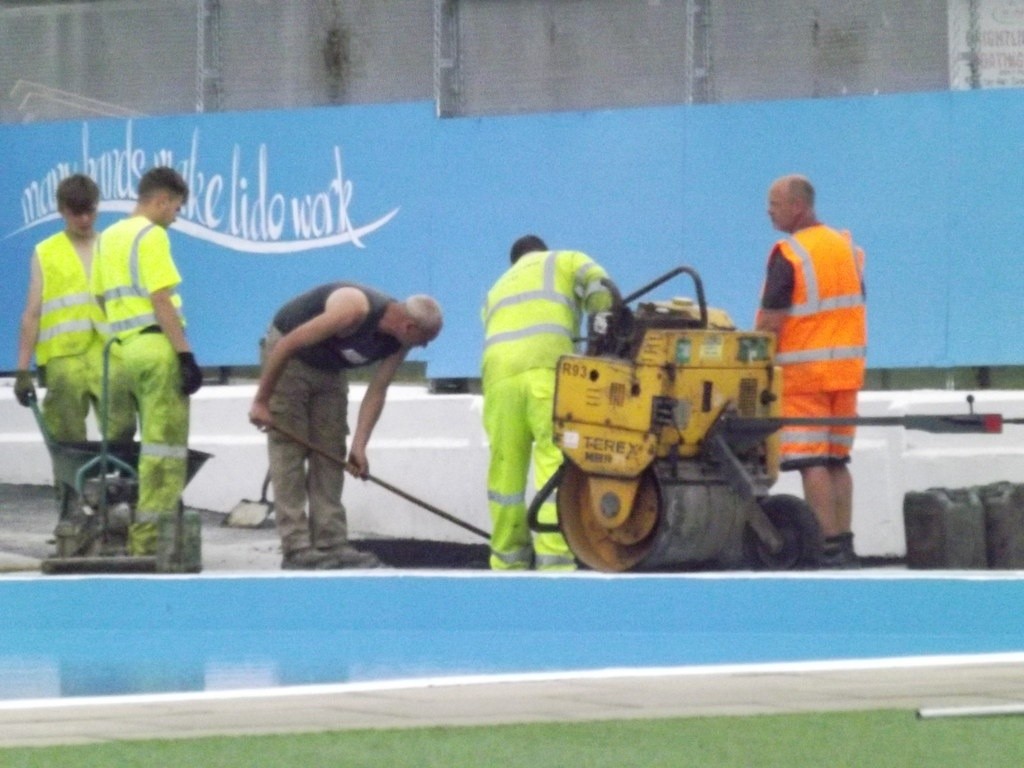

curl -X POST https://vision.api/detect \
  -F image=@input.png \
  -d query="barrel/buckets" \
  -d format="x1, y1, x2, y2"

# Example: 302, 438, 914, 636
903, 482, 1024, 568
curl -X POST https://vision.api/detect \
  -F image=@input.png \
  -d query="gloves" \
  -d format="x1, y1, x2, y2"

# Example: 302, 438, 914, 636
15, 372, 37, 407
178, 351, 203, 395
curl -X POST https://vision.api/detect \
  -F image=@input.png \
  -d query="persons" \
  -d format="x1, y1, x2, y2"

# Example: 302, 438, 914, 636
752, 175, 867, 570
479, 234, 612, 573
86, 165, 202, 558
13, 174, 107, 444
249, 282, 443, 570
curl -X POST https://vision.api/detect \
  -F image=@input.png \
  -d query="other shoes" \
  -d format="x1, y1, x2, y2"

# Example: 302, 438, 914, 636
281, 550, 340, 570
327, 544, 379, 568
821, 544, 861, 570
